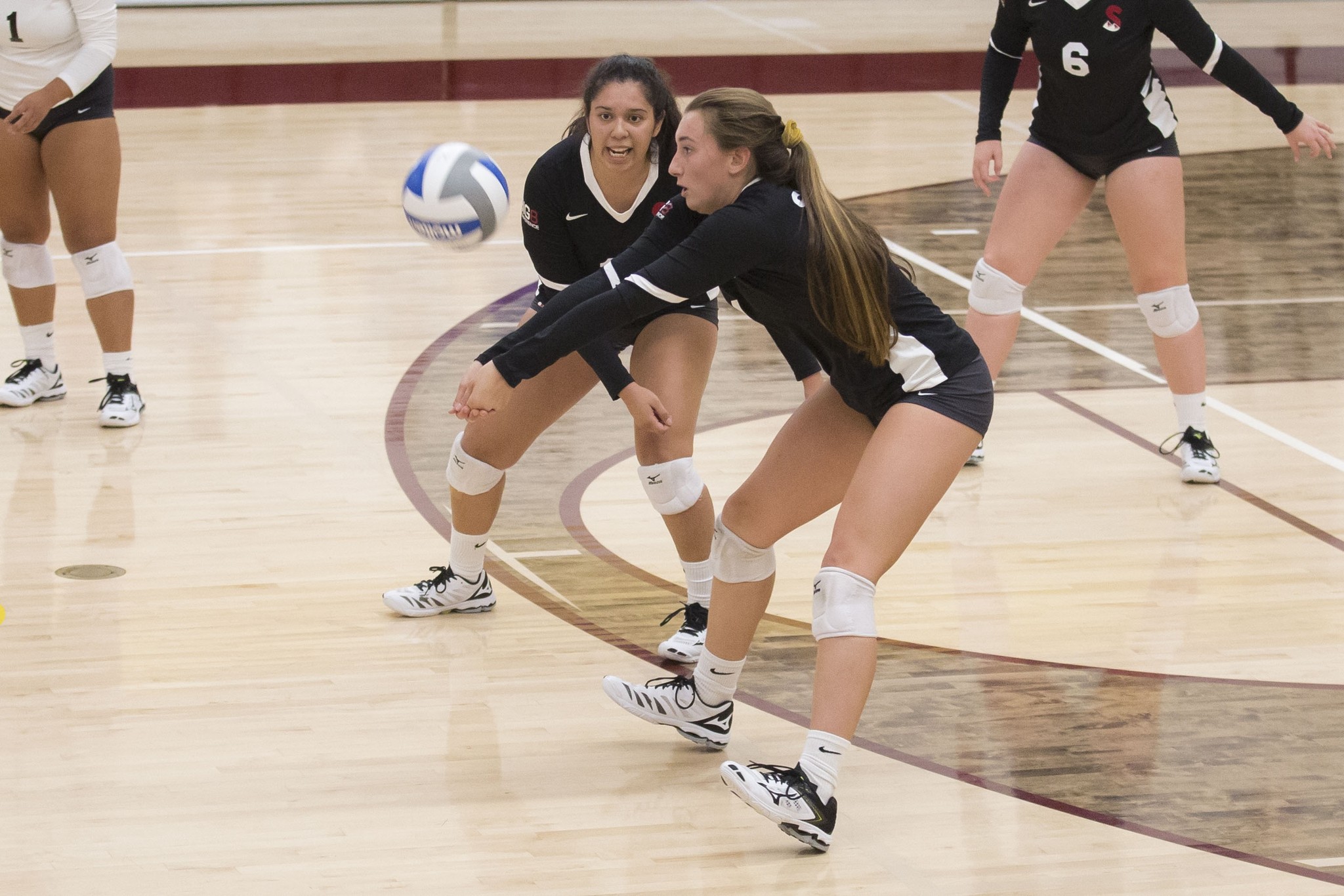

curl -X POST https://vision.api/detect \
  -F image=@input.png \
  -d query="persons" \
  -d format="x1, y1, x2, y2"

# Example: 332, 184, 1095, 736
958, 1, 1335, 484
449, 84, 994, 852
383, 53, 718, 665
0, 0, 146, 428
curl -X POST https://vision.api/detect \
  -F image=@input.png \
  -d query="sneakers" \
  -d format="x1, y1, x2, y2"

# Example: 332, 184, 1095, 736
966, 440, 984, 465
601, 673, 733, 751
381, 563, 497, 618
720, 759, 837, 852
96, 372, 144, 427
658, 602, 708, 663
1180, 425, 1219, 483
0, 358, 67, 407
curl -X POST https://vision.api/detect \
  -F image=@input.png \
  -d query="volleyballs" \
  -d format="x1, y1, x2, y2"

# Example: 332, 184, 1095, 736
401, 141, 509, 252
